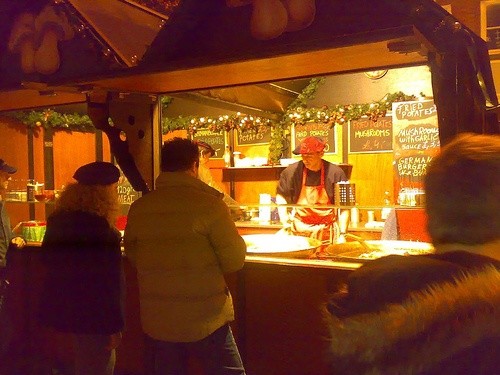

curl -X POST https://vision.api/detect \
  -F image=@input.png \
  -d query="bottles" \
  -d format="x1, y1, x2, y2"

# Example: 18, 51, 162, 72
224, 144, 231, 167
381, 191, 392, 207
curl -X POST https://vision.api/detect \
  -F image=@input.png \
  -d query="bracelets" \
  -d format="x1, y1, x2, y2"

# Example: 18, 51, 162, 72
339, 232, 346, 235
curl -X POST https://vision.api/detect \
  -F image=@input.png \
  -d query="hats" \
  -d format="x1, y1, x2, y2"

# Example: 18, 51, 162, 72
293, 136, 326, 155
0, 159, 17, 174
73, 162, 120, 184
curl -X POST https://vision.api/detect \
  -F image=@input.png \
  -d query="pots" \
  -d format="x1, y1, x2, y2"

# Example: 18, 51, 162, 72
326, 240, 438, 262
240, 235, 321, 259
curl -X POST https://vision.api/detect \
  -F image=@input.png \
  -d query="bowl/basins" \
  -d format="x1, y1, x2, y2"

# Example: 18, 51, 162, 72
35, 194, 45, 202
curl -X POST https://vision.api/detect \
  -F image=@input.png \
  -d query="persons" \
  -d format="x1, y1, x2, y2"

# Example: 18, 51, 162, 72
197, 142, 225, 197
36, 162, 125, 375
322, 133, 500, 375
123, 137, 247, 375
0, 158, 26, 311
276, 137, 350, 246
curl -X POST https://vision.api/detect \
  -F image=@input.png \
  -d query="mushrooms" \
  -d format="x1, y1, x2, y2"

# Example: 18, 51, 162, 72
7, 2, 76, 75
225, 0, 316, 41
